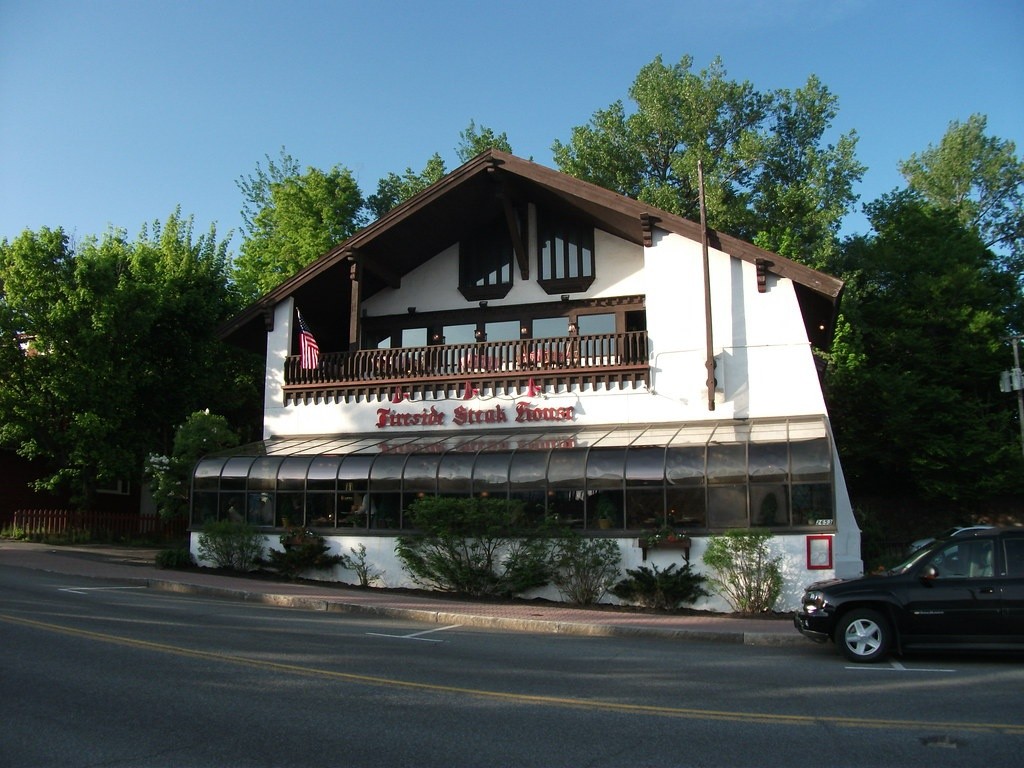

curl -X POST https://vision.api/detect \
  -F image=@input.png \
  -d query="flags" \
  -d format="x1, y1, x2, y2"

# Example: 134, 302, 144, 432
296, 308, 323, 371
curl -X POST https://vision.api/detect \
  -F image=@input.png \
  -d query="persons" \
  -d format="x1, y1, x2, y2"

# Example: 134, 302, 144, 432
347, 489, 382, 528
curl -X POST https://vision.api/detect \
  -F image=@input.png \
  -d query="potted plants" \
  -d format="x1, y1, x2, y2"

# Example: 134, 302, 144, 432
595, 498, 616, 530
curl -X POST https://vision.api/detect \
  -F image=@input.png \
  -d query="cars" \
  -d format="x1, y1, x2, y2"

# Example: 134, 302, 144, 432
909, 523, 999, 556
792, 526, 1024, 666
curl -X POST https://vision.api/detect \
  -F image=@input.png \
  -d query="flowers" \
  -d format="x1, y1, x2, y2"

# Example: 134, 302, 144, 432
280, 528, 326, 545
642, 527, 689, 547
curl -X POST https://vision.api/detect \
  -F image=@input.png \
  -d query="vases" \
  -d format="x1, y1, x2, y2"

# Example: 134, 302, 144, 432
639, 539, 691, 549
286, 536, 322, 546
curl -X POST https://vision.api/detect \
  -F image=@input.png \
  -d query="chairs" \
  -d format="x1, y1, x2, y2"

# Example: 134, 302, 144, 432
974, 542, 994, 578
962, 547, 981, 578
